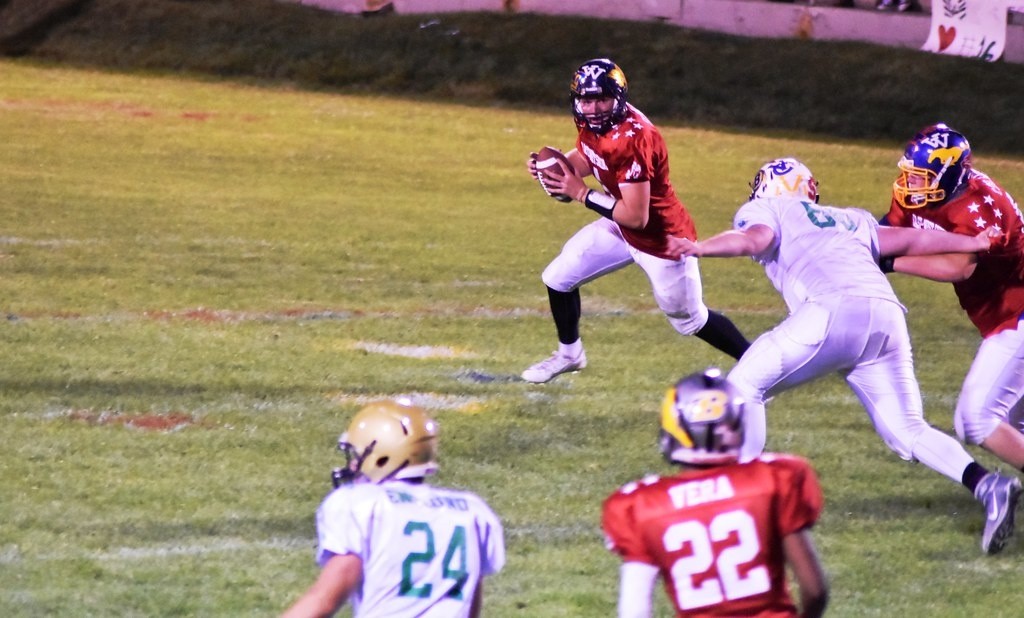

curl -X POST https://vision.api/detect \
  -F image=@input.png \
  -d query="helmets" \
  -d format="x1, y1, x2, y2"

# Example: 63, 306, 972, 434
892, 123, 973, 210
331, 400, 439, 490
748, 157, 820, 203
568, 58, 629, 132
656, 367, 747, 469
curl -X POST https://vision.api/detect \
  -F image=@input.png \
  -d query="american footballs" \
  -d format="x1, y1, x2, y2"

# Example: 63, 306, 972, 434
536, 146, 574, 203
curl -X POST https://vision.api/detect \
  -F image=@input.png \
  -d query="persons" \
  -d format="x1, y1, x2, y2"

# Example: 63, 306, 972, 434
878, 125, 1024, 475
665, 158, 1020, 552
603, 372, 831, 616
274, 400, 506, 617
519, 58, 751, 384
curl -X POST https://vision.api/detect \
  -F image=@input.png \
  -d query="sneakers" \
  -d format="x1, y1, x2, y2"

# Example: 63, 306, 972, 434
520, 348, 587, 384
974, 471, 1023, 556
